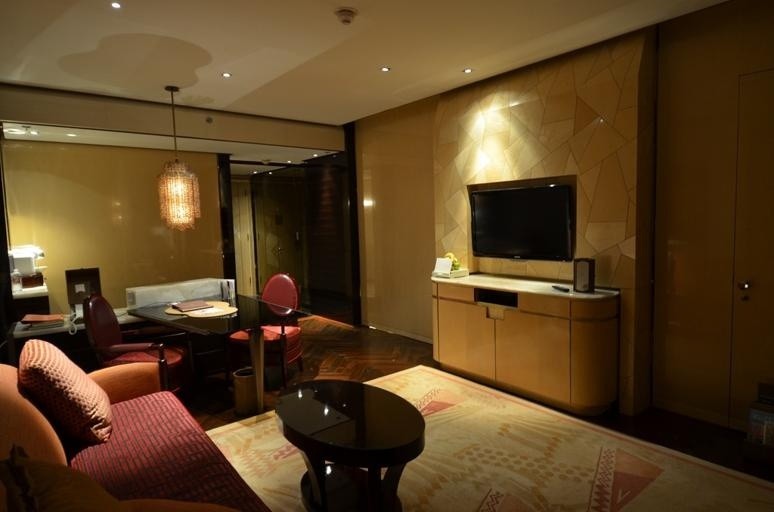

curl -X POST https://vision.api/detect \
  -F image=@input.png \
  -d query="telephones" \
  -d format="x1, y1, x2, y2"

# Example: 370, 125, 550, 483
75, 303, 84, 319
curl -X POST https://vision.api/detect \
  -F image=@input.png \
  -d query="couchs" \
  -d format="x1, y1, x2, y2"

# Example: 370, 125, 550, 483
0, 359, 272, 511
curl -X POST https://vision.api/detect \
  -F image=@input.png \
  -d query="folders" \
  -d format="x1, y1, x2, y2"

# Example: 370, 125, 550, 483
171, 300, 213, 313
19, 313, 64, 325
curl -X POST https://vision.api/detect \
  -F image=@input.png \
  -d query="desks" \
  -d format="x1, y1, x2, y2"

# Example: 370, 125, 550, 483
275, 379, 425, 512
127, 291, 313, 412
13, 308, 156, 343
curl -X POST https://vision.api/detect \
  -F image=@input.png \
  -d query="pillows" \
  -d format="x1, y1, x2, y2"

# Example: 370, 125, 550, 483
16, 338, 113, 442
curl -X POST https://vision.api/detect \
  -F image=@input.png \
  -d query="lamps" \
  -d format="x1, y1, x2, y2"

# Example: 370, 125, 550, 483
158, 84, 203, 232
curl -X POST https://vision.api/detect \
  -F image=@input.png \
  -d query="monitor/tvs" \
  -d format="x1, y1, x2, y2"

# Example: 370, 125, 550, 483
471, 184, 572, 261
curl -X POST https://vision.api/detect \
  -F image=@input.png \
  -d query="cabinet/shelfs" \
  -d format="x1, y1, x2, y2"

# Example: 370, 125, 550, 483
11, 278, 49, 315
431, 272, 619, 417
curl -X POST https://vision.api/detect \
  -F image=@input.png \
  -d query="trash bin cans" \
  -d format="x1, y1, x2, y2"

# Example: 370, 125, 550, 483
232, 366, 260, 416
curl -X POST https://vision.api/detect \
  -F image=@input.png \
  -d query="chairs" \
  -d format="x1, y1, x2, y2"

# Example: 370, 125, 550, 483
227, 271, 307, 389
82, 291, 190, 403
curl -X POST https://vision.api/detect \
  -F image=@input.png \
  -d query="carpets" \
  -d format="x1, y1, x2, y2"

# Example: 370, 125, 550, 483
205, 361, 774, 510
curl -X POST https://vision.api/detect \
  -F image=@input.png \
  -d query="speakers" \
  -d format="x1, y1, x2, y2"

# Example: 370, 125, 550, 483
573, 258, 595, 293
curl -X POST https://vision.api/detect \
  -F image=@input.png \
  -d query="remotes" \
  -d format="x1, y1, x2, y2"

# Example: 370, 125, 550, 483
552, 286, 569, 292
